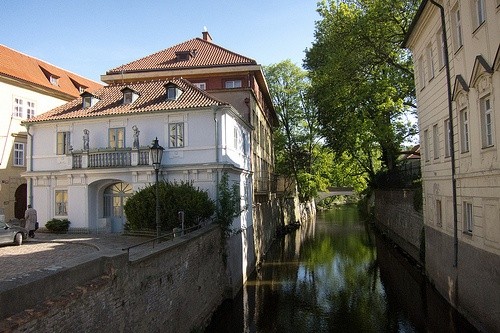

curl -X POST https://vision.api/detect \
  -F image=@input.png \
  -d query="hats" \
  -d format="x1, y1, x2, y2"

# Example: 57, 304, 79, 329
28, 204, 32, 208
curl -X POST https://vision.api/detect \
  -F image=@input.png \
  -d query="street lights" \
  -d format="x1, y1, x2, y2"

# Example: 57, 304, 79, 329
149, 137, 165, 245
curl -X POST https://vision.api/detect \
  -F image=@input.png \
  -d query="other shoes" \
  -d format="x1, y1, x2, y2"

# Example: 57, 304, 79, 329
32, 235, 35, 238
28, 234, 31, 237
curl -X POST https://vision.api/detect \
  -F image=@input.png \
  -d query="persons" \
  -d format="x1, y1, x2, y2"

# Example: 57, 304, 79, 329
131, 126, 140, 148
83, 129, 89, 149
24, 205, 38, 238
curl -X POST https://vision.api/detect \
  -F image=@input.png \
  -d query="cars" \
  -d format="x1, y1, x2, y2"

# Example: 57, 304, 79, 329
0, 219, 29, 246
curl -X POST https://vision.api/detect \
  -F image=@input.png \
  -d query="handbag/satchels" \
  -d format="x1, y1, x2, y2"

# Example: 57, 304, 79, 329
34, 222, 38, 231
